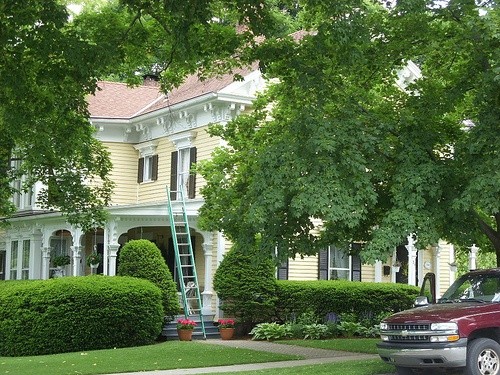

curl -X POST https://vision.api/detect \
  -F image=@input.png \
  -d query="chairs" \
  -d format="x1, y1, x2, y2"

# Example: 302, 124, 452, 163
175, 281, 196, 315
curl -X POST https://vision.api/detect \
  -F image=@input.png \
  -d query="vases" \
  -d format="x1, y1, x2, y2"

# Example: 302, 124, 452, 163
220, 328, 235, 340
176, 329, 194, 341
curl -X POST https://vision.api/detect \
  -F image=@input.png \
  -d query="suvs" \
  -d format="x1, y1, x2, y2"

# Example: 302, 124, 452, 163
376, 268, 500, 375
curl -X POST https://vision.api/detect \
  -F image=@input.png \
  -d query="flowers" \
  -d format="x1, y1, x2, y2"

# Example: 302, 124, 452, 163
176, 318, 197, 329
218, 319, 234, 328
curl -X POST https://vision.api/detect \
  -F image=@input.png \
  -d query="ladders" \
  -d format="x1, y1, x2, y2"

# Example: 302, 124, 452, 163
164, 184, 207, 341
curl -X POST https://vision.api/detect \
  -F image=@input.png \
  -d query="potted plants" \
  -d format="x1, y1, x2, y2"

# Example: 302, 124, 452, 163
86, 251, 102, 269
393, 261, 402, 273
52, 255, 70, 270
